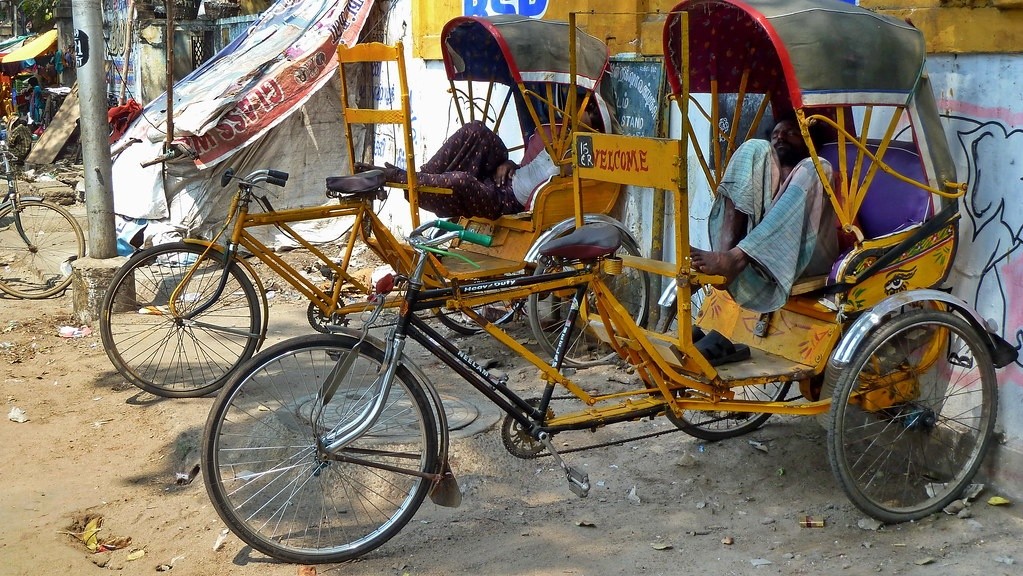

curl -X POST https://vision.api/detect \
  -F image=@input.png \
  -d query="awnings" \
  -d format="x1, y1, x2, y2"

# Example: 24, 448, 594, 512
0, 29, 59, 64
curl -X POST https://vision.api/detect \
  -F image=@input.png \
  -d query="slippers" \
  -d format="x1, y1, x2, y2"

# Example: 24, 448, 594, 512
691, 324, 705, 344
694, 329, 750, 366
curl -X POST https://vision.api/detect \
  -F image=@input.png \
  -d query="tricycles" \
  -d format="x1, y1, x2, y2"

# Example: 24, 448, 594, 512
97, 12, 653, 404
195, 1, 1022, 566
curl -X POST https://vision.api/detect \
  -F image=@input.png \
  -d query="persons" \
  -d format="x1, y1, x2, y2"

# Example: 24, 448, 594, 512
354, 95, 600, 218
24, 76, 44, 127
0, 115, 34, 167
687, 113, 841, 313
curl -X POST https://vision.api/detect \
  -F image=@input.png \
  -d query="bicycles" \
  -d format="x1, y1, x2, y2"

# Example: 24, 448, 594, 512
0, 149, 86, 298
